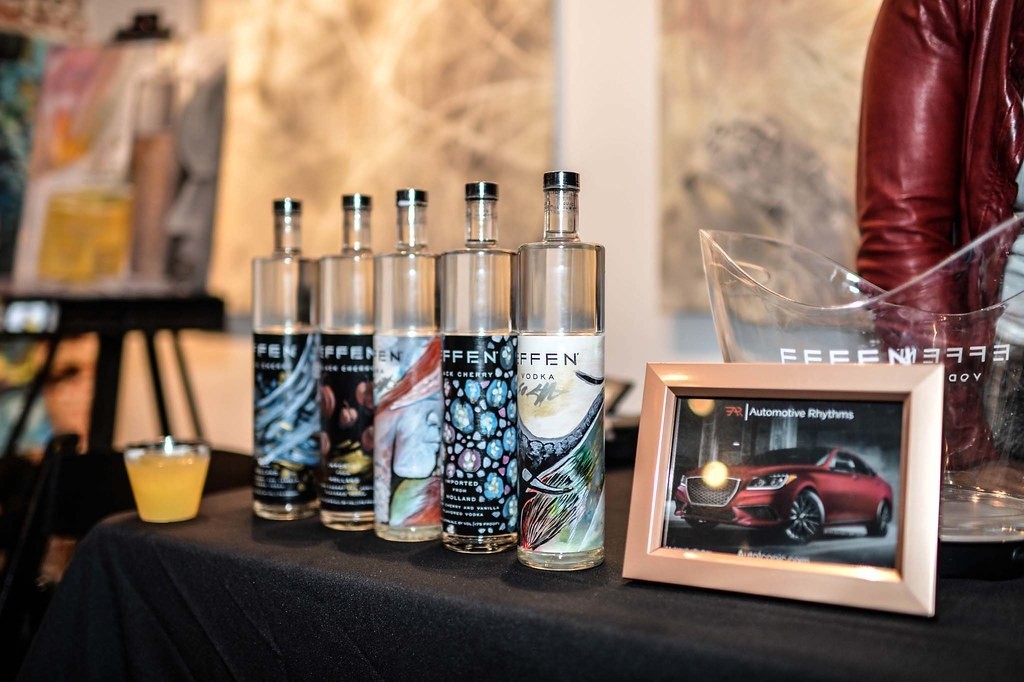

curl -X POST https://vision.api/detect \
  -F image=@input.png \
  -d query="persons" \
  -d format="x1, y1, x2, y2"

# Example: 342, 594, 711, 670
855, 0, 1024, 499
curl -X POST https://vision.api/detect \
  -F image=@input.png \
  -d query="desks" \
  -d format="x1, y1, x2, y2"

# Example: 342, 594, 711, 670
44, 487, 1024, 682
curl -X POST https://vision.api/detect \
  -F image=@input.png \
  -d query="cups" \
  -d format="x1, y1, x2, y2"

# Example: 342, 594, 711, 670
123, 438, 210, 524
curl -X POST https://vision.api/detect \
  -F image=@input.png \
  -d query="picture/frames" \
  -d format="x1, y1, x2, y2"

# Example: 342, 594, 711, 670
621, 362, 947, 619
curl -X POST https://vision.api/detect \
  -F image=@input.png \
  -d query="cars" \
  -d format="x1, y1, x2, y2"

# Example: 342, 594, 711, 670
675, 447, 893, 547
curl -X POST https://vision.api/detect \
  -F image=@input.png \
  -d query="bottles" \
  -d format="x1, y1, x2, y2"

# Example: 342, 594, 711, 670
435, 180, 518, 555
373, 189, 442, 543
318, 194, 376, 532
251, 199, 322, 521
510, 172, 608, 572
